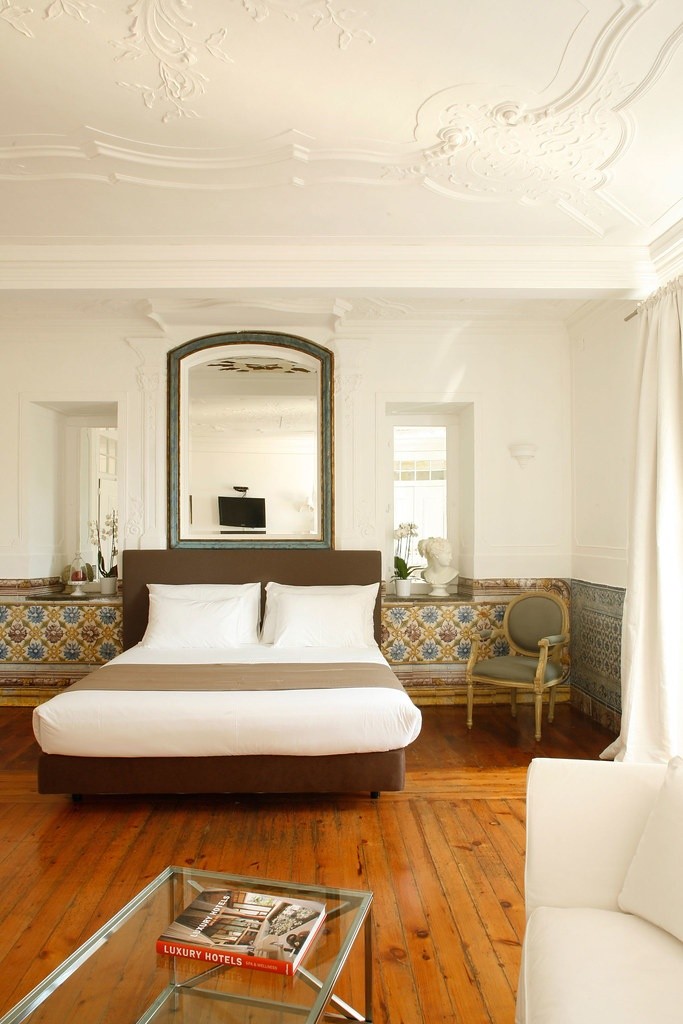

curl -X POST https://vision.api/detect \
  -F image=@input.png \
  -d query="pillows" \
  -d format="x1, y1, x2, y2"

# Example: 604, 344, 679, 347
146, 594, 262, 643
141, 583, 262, 644
261, 581, 381, 644
265, 588, 379, 647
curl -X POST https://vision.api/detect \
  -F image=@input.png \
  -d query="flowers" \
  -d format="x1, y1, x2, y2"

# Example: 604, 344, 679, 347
89, 511, 120, 577
389, 522, 424, 581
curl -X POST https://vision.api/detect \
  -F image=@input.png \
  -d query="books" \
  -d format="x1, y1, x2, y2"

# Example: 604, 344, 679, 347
155, 886, 327, 975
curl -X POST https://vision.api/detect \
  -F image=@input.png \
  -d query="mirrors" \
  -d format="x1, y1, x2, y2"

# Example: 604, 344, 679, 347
166, 330, 341, 546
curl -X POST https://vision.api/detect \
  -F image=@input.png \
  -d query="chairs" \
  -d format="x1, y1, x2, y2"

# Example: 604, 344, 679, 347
466, 592, 571, 741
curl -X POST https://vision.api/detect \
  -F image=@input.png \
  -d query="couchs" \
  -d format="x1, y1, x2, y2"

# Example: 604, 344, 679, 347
514, 755, 683, 1022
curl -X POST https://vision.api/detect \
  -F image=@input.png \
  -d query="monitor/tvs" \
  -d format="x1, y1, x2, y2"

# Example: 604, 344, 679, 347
218, 497, 266, 528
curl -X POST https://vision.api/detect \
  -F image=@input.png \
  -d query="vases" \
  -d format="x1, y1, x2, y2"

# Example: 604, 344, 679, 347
395, 577, 414, 596
100, 574, 117, 595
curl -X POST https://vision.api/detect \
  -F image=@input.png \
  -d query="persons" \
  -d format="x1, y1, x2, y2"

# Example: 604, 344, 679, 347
419, 537, 460, 583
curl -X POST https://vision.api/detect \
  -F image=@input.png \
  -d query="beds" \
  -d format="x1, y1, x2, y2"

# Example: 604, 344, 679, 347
34, 549, 423, 810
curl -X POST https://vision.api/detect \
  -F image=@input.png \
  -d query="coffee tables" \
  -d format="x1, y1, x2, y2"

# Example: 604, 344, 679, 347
1, 865, 375, 1024
